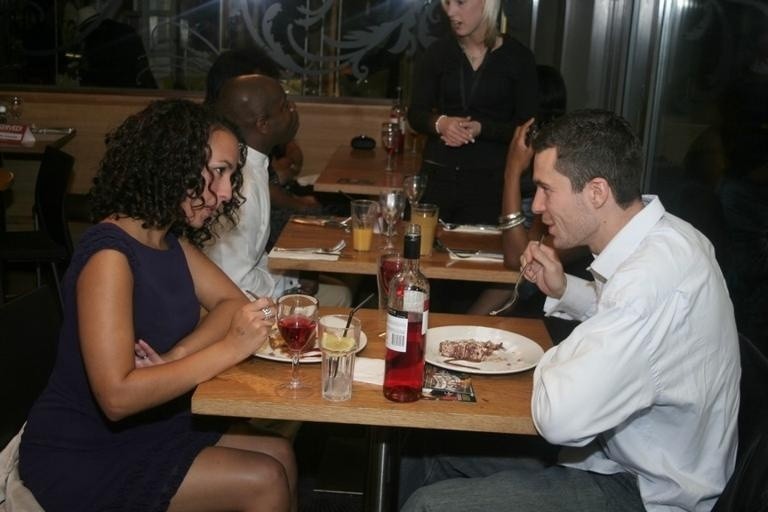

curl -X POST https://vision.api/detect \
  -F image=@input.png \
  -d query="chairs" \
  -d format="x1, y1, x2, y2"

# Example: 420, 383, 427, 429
0, 143, 81, 334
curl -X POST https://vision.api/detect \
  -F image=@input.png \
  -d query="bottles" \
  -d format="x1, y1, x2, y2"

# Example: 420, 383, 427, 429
391, 87, 405, 144
382, 222, 427, 403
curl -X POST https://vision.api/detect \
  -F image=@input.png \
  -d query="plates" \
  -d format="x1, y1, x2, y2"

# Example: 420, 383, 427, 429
425, 324, 544, 375
254, 314, 366, 365
350, 136, 375, 150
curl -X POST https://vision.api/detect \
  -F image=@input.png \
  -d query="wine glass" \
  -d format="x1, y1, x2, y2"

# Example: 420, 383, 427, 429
378, 250, 406, 341
380, 121, 401, 174
405, 107, 420, 154
277, 294, 319, 400
378, 192, 403, 253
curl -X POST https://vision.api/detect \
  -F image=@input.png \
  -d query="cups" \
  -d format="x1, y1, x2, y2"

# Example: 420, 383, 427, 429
409, 201, 438, 259
403, 174, 427, 208
350, 199, 377, 253
6, 95, 24, 120
317, 313, 361, 404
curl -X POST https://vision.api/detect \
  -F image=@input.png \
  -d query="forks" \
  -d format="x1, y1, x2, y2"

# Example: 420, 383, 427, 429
444, 247, 505, 262
275, 240, 347, 254
486, 233, 547, 315
